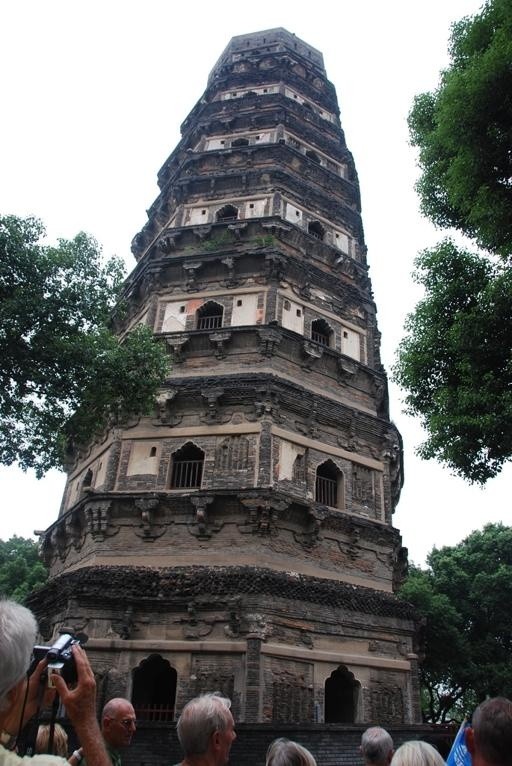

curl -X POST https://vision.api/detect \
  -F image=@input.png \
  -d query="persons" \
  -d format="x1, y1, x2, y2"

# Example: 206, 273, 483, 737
173, 690, 237, 766
265, 737, 317, 766
465, 696, 512, 766
358, 726, 395, 765
1, 602, 138, 766
390, 739, 449, 766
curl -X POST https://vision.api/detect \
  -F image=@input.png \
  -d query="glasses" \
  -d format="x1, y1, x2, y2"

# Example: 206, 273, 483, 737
111, 718, 137, 728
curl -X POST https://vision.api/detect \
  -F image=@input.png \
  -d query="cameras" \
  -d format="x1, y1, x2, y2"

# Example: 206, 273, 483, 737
32, 634, 82, 690
